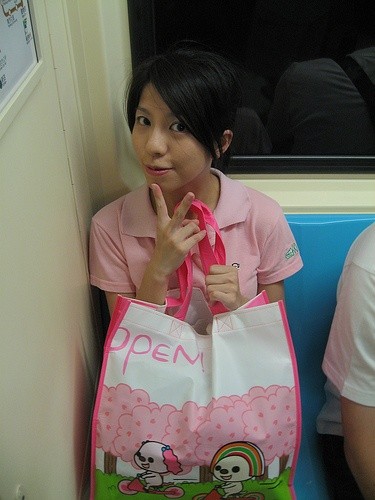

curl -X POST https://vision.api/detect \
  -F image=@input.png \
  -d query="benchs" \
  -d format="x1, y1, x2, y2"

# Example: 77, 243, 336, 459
276, 212, 374, 500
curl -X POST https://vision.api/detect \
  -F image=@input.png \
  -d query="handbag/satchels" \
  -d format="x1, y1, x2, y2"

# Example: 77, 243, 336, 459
91, 199, 303, 500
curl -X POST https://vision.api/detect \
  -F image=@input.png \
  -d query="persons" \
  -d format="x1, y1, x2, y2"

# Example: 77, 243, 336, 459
314, 220, 375, 500
344, 23, 375, 127
262, 33, 375, 168
230, 107, 274, 166
88, 32, 305, 334
